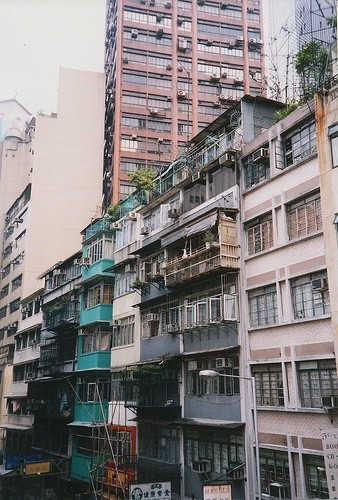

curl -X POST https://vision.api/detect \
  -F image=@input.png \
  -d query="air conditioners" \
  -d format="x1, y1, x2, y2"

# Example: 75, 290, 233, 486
321, 396, 335, 409
125, 212, 137, 220
269, 483, 291, 500
168, 324, 177, 332
110, 223, 121, 231
140, 227, 152, 235
218, 153, 235, 167
188, 360, 204, 370
173, 166, 192, 189
190, 460, 212, 474
312, 277, 328, 291
252, 147, 269, 163
215, 357, 233, 368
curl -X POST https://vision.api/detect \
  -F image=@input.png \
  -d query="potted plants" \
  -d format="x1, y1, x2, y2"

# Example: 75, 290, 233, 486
146, 313, 160, 321
202, 230, 216, 250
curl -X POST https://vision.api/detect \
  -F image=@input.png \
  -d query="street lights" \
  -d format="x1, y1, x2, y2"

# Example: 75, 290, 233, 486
198, 370, 263, 500
